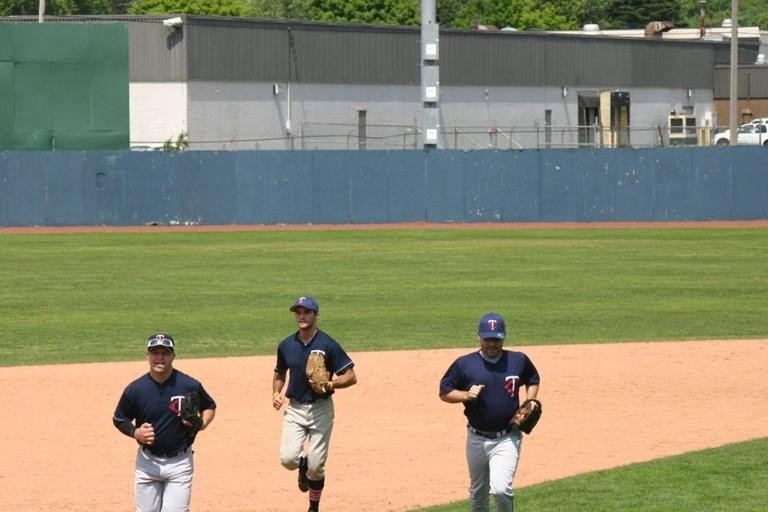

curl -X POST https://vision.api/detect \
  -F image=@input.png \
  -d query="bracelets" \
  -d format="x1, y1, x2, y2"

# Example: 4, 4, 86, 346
328, 381, 333, 391
272, 392, 279, 399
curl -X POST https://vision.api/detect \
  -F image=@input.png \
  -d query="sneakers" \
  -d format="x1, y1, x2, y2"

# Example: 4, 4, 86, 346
297, 455, 310, 493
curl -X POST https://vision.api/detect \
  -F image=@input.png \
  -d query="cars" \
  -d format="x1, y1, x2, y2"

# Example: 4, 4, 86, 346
712, 123, 768, 147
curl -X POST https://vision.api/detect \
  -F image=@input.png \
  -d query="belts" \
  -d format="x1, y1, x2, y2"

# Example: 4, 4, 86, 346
466, 425, 513, 440
289, 396, 328, 406
139, 444, 187, 459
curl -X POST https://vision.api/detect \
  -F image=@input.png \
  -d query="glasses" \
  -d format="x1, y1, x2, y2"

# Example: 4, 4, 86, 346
146, 338, 174, 353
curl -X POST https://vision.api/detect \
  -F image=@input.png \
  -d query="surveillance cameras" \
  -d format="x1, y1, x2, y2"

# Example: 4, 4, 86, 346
162, 17, 184, 28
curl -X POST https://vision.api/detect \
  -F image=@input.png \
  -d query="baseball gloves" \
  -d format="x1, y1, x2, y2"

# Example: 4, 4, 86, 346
508, 399, 541, 435
178, 392, 203, 429
306, 353, 335, 397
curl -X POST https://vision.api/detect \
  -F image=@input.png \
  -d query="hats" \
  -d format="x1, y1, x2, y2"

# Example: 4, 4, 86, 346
477, 312, 507, 341
288, 296, 319, 313
146, 332, 175, 355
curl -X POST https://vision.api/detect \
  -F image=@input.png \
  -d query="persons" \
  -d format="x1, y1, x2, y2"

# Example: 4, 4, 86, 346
112, 334, 217, 512
438, 311, 542, 511
271, 296, 357, 512
